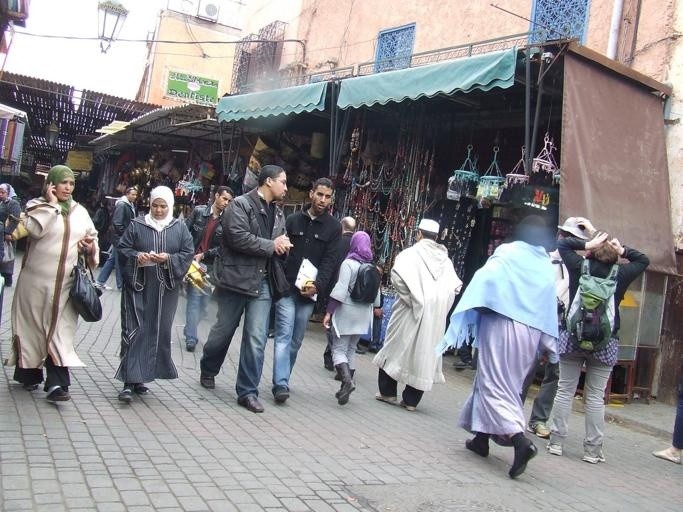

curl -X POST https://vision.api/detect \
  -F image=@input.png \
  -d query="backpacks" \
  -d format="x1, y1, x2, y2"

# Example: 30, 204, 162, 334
566, 259, 620, 352
348, 258, 382, 303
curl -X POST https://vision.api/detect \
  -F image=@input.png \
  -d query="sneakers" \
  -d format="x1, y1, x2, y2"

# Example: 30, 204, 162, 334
453, 360, 683, 477
94, 284, 112, 291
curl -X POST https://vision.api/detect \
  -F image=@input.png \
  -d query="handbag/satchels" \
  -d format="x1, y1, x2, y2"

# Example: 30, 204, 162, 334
70, 262, 102, 322
267, 255, 291, 299
5, 213, 28, 243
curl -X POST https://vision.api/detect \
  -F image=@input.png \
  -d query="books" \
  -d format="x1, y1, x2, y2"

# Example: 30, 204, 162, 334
294, 257, 319, 303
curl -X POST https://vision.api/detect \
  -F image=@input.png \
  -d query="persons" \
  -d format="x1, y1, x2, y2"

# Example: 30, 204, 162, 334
0, 183, 234, 351
440, 211, 560, 478
271, 176, 343, 402
518, 211, 598, 439
544, 230, 650, 465
321, 216, 359, 371
201, 165, 295, 413
114, 186, 194, 400
0, 163, 100, 400
370, 218, 464, 410
322, 230, 385, 405
651, 382, 682, 466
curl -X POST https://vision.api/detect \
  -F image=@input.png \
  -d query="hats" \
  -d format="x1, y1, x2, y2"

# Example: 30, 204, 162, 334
418, 219, 440, 234
557, 217, 590, 240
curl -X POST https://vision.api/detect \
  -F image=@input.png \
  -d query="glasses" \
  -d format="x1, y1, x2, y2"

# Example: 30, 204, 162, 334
126, 186, 139, 193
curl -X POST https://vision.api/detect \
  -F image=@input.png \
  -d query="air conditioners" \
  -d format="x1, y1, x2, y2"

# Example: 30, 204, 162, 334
197, 0, 221, 21
